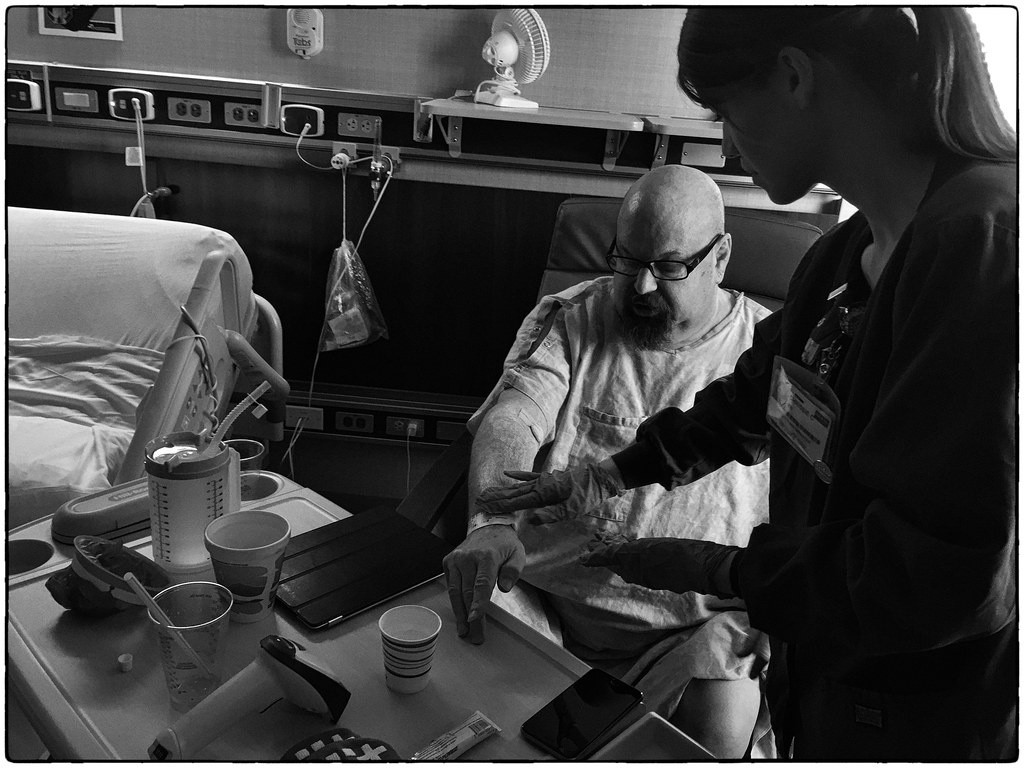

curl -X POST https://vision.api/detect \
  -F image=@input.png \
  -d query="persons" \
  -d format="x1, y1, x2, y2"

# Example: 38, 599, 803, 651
443, 165, 772, 759
476, 4, 1019, 766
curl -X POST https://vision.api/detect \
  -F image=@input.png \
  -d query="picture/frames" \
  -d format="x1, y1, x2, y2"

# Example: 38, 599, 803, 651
38, 7, 125, 42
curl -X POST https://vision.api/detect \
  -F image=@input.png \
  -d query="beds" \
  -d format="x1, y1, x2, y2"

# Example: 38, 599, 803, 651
5, 205, 289, 530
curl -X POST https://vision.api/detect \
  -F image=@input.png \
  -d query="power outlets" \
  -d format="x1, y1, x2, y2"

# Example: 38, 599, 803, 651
224, 102, 268, 129
167, 96, 211, 123
338, 113, 380, 138
385, 416, 424, 437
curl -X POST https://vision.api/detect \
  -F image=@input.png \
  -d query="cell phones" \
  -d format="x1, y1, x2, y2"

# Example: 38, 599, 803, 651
521, 667, 644, 761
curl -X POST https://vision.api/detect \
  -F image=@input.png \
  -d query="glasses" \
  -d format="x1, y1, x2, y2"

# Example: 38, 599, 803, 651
605, 234, 726, 281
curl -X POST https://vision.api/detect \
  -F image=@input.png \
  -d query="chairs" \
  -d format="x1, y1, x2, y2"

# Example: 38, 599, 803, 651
536, 193, 823, 316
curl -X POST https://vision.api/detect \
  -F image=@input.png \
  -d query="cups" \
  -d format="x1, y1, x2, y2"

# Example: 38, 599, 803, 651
147, 580, 233, 711
223, 439, 265, 471
202, 510, 290, 623
143, 382, 272, 576
378, 605, 441, 695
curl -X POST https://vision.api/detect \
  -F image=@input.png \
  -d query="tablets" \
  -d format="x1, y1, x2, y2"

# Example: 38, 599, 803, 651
273, 504, 457, 630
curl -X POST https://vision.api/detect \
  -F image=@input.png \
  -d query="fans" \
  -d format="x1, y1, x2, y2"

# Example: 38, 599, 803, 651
473, 8, 551, 108
